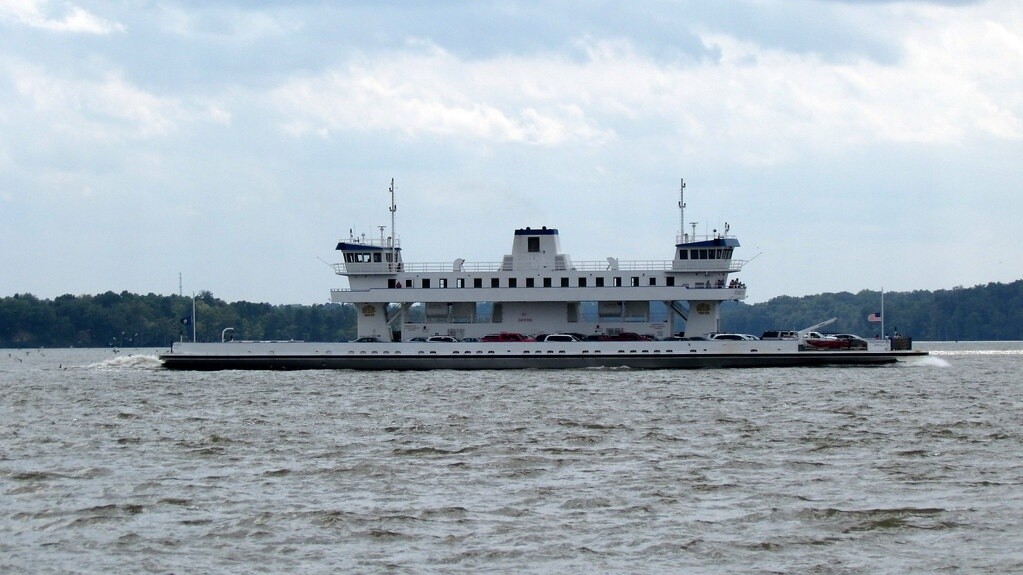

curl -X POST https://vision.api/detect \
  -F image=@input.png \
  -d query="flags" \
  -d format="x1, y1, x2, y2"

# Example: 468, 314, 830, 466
867, 312, 882, 322
179, 315, 193, 327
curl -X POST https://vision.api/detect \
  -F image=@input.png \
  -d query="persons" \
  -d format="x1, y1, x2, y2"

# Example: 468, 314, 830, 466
883, 333, 890, 340
875, 334, 880, 340
716, 276, 746, 291
704, 280, 712, 289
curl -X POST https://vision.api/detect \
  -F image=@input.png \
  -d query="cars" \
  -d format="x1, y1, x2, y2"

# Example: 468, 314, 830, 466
341, 330, 868, 348
